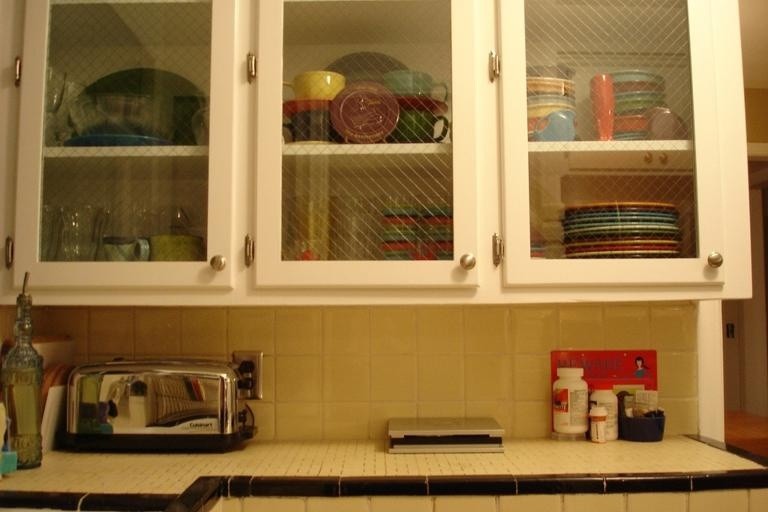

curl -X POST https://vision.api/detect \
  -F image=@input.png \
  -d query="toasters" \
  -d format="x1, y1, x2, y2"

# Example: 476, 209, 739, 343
60, 356, 257, 454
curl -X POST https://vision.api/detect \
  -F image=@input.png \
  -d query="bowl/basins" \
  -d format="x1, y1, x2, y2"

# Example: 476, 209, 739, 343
382, 202, 454, 260
148, 234, 204, 261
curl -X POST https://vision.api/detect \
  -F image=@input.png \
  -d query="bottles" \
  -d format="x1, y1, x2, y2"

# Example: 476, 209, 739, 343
587, 384, 618, 444
551, 367, 589, 433
0, 293, 44, 469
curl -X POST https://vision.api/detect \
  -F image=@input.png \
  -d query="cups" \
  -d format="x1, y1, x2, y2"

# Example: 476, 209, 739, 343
590, 73, 617, 141
534, 107, 578, 142
282, 70, 347, 144
41, 203, 111, 261
44, 66, 67, 114
383, 70, 449, 142
102, 236, 150, 261
643, 106, 682, 140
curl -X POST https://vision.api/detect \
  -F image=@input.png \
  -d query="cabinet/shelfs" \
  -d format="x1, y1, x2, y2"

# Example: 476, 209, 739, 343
0, 1, 241, 310
242, 1, 483, 309
483, 0, 755, 306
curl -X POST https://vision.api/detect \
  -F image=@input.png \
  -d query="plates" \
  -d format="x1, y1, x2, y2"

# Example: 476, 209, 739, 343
609, 69, 666, 140
63, 68, 207, 147
527, 61, 579, 141
330, 80, 401, 142
325, 52, 410, 84
563, 199, 682, 257
